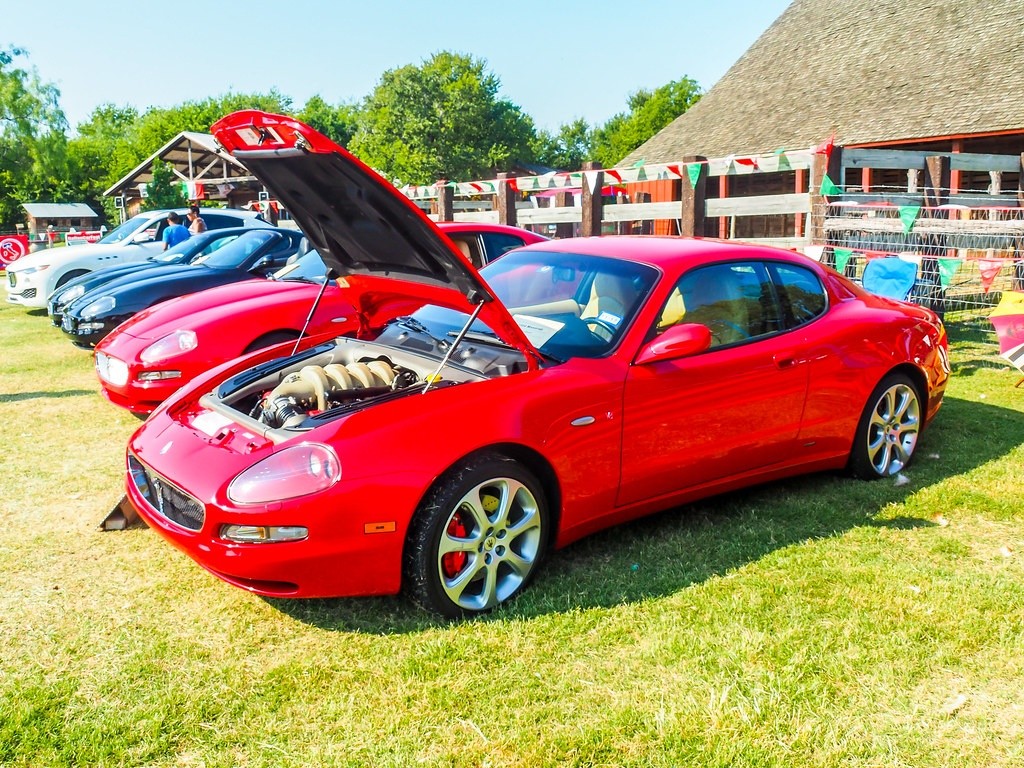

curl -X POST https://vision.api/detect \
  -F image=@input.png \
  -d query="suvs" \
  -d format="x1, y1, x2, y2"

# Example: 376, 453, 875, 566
5, 209, 276, 308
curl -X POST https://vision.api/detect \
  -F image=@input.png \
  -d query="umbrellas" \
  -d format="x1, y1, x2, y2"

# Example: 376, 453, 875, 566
986, 288, 1024, 387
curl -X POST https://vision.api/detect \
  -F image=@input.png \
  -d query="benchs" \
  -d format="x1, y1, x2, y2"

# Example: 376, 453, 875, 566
658, 286, 822, 336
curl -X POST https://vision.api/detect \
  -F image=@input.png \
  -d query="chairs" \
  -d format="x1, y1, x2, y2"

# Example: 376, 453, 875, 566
580, 272, 637, 342
674, 268, 749, 348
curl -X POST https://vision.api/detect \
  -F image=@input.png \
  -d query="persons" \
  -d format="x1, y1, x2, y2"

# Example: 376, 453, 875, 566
162, 211, 192, 250
188, 204, 207, 235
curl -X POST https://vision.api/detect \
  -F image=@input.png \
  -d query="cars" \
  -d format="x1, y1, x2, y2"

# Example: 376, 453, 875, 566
93, 212, 553, 422
47, 227, 307, 343
125, 109, 950, 618
